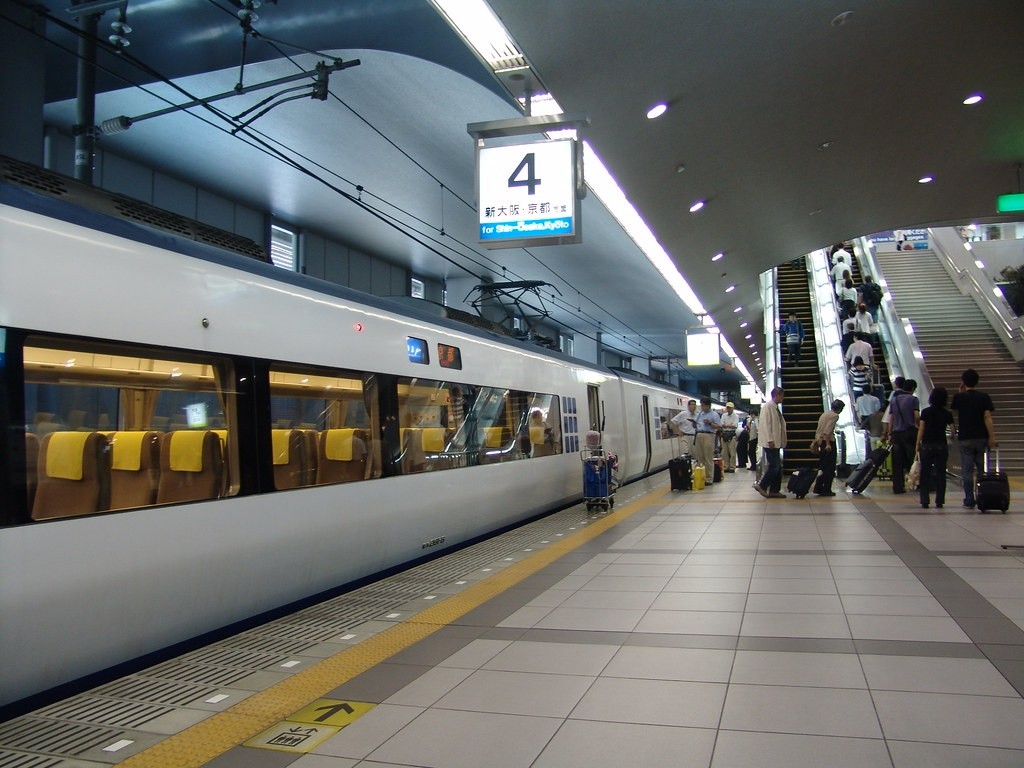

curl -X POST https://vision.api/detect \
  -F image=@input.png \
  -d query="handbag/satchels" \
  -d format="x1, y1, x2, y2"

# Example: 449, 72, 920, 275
905, 452, 921, 491
695, 459, 724, 490
787, 334, 800, 344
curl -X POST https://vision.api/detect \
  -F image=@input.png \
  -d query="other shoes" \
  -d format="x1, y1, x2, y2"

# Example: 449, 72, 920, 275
754, 484, 769, 498
963, 502, 973, 509
736, 464, 746, 468
936, 503, 943, 508
747, 467, 755, 471
769, 492, 786, 498
922, 503, 929, 508
812, 487, 836, 496
724, 469, 734, 473
787, 451, 831, 499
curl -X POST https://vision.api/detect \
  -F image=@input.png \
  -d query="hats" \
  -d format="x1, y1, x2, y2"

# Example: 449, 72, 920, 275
726, 402, 734, 407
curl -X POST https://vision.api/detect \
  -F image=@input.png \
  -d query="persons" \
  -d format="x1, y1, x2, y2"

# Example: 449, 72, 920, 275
527, 410, 556, 438
657, 396, 759, 486
771, 228, 958, 520
949, 368, 996, 508
754, 385, 790, 500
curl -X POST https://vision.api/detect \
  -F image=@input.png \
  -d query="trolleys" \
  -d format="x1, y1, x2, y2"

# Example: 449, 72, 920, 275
581, 448, 624, 512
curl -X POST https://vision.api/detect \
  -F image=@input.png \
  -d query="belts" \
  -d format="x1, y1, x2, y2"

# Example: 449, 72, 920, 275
684, 433, 694, 436
697, 431, 713, 433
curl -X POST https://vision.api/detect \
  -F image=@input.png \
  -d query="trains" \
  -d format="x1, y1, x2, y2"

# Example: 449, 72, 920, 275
1, 152, 751, 700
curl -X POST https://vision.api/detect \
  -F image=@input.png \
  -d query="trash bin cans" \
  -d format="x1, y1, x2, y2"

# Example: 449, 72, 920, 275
583, 456, 611, 500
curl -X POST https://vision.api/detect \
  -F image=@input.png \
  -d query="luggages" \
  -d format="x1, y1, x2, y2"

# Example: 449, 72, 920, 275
873, 367, 885, 408
877, 440, 893, 480
976, 445, 1011, 514
844, 444, 894, 494
666, 438, 693, 491
